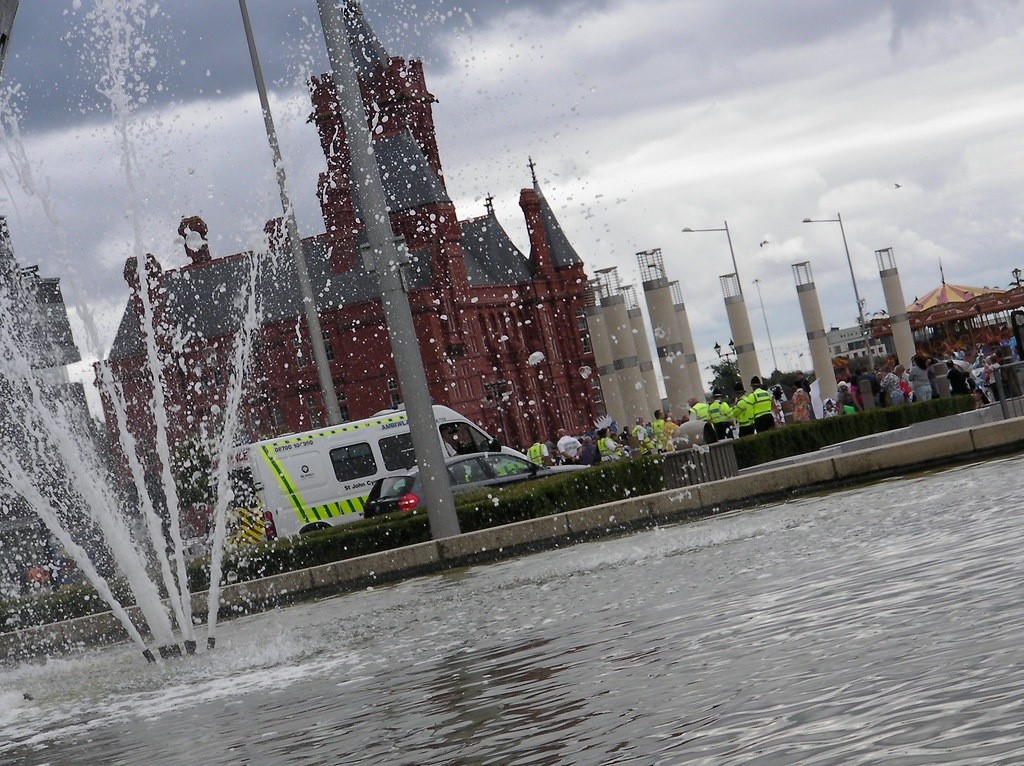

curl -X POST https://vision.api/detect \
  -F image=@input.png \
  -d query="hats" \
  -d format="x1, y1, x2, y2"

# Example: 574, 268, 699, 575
714, 389, 722, 396
734, 383, 744, 392
795, 370, 803, 375
751, 376, 760, 385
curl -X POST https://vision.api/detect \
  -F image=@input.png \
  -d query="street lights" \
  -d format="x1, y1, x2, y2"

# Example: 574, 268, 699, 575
802, 212, 877, 371
751, 277, 779, 377
682, 220, 744, 295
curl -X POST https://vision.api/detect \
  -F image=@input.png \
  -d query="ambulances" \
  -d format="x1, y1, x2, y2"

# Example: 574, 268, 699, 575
214, 404, 534, 546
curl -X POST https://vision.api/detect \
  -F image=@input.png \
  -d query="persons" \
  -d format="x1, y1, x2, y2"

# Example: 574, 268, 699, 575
775, 328, 1020, 424
523, 375, 774, 466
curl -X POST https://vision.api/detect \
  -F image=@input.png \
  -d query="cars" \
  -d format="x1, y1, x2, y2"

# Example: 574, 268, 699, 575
363, 451, 596, 518
